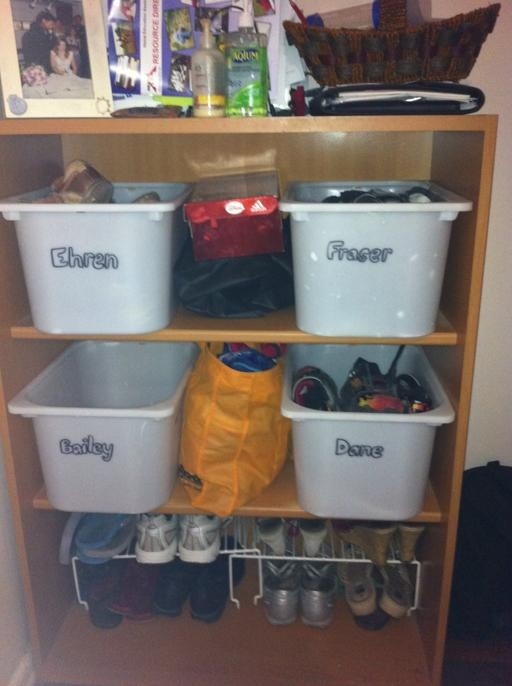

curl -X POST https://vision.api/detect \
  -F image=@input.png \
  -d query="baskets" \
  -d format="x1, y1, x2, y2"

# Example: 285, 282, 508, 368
281, 2, 502, 87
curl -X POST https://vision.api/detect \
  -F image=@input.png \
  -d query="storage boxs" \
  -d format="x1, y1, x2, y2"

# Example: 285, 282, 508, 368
3, 339, 204, 517
277, 175, 474, 339
183, 167, 291, 264
2, 180, 200, 336
278, 341, 457, 528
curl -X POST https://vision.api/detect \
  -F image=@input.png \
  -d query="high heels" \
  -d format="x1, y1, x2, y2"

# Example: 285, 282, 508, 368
332, 517, 396, 567
258, 516, 286, 556
298, 517, 326, 558
396, 521, 426, 563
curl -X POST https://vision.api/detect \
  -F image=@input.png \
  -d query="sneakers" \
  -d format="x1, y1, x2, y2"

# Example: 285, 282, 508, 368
53, 510, 245, 633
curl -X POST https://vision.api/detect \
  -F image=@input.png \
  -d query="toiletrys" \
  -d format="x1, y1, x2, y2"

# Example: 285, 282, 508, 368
226, 0, 268, 117
190, 6, 226, 117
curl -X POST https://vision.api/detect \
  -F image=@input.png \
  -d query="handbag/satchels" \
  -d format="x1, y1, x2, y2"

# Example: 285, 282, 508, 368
178, 337, 292, 518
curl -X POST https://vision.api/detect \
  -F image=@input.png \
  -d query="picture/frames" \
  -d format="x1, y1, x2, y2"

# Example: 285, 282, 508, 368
0, 0, 114, 120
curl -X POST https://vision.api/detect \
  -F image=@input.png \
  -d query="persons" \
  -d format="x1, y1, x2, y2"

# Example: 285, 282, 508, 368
21, 10, 94, 99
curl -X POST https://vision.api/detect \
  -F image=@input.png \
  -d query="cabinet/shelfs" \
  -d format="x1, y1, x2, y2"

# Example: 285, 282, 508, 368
0, 105, 501, 686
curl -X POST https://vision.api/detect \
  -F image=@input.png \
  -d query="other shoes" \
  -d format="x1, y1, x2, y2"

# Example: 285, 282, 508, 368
259, 559, 413, 632
